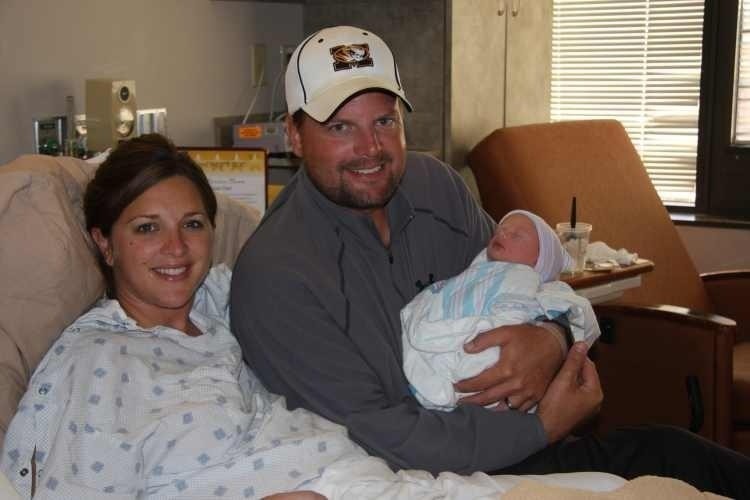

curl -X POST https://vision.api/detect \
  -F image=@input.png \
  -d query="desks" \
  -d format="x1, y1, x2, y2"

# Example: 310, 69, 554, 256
555, 250, 655, 306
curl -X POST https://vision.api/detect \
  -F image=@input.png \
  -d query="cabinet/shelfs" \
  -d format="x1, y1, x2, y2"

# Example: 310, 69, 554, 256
302, 0, 553, 166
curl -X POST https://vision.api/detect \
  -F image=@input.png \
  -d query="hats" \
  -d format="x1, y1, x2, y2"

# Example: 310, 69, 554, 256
498, 209, 565, 283
283, 24, 414, 124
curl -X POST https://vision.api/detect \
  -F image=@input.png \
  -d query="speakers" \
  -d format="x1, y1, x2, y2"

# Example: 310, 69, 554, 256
85, 78, 137, 154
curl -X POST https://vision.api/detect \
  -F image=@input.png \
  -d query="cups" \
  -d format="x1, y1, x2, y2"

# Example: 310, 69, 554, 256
556, 222, 591, 274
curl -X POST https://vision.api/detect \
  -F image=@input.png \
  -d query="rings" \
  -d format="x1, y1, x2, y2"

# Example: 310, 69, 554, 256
504, 398, 518, 410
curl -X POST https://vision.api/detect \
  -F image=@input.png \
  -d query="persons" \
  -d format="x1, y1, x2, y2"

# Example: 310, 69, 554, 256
228, 25, 750, 499
484, 210, 572, 285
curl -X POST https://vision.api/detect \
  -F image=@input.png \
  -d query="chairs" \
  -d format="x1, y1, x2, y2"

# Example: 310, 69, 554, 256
469, 117, 750, 456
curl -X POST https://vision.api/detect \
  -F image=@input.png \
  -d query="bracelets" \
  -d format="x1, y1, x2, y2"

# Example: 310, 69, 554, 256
527, 316, 570, 360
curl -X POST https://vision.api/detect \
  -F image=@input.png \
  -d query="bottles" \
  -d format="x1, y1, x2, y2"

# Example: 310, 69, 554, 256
63, 96, 77, 158
